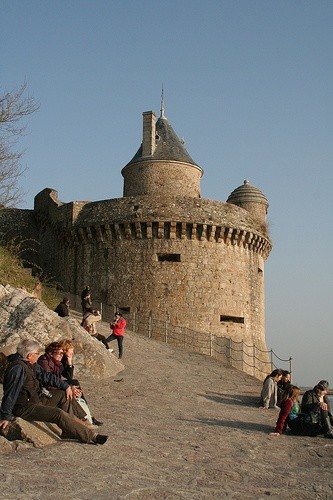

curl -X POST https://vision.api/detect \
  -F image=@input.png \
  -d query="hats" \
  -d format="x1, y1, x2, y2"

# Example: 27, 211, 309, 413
318, 380, 329, 390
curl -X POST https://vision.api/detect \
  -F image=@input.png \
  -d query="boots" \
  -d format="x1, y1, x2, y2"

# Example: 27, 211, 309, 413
321, 416, 333, 438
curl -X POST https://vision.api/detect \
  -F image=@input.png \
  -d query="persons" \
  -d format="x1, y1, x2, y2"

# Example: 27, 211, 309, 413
270, 384, 332, 438
36, 342, 89, 426
1, 337, 109, 445
54, 297, 70, 316
81, 307, 114, 353
82, 293, 92, 313
82, 285, 90, 300
260, 369, 283, 409
300, 380, 332, 428
106, 312, 126, 358
275, 371, 294, 407
58, 340, 103, 425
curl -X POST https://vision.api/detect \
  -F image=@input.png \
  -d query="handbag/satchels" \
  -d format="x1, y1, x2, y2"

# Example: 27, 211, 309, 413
288, 414, 319, 437
76, 398, 92, 424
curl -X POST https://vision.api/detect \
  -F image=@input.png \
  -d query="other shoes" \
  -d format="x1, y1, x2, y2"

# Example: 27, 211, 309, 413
92, 435, 109, 445
61, 432, 78, 439
92, 417, 103, 426
108, 349, 114, 353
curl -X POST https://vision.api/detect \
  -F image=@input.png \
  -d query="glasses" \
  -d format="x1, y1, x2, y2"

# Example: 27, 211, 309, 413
317, 386, 324, 391
54, 351, 63, 357
